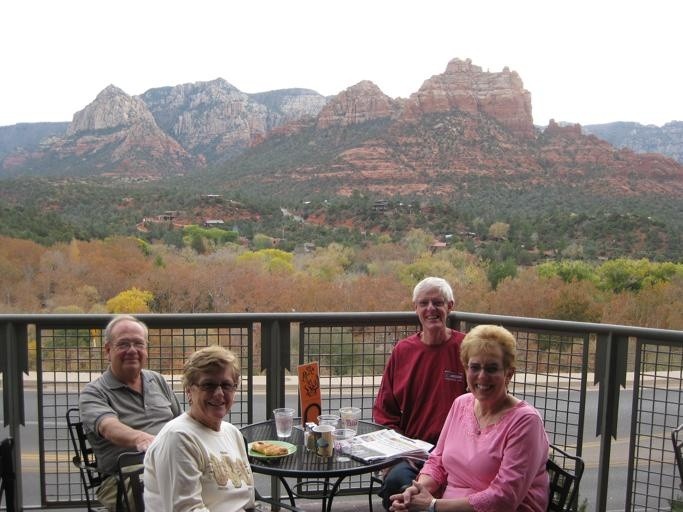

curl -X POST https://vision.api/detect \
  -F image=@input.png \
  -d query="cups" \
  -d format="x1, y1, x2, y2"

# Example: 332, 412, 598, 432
272, 407, 361, 462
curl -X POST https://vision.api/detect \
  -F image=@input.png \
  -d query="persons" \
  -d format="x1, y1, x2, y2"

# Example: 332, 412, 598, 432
143, 345, 256, 512
388, 325, 551, 512
79, 315, 182, 512
374, 277, 466, 512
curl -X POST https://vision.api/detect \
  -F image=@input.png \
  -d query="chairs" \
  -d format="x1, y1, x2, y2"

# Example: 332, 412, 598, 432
115, 450, 146, 512
670, 424, 682, 474
66, 408, 102, 512
546, 445, 585, 511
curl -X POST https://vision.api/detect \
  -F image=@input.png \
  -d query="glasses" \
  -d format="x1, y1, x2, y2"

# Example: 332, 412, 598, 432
192, 381, 238, 392
467, 364, 507, 374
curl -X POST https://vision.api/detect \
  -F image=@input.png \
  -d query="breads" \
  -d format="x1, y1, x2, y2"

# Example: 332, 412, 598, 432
252, 441, 288, 456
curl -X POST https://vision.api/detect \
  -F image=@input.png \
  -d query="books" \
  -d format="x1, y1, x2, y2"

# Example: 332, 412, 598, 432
340, 429, 435, 464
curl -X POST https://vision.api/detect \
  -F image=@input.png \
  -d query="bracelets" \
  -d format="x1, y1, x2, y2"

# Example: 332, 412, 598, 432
430, 498, 436, 512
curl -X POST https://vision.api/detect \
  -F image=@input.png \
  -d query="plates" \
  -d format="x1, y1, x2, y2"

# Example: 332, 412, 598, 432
247, 440, 297, 462
